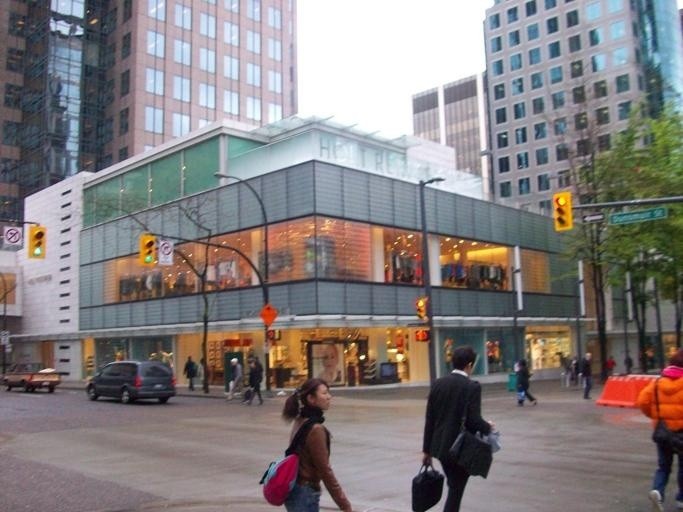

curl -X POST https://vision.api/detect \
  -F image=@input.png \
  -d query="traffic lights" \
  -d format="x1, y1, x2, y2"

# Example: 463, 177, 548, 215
414, 328, 430, 343
551, 190, 574, 233
414, 296, 428, 319
27, 225, 47, 260
137, 233, 157, 265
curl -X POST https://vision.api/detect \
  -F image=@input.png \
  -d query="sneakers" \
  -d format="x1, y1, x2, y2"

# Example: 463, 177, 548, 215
647, 489, 663, 512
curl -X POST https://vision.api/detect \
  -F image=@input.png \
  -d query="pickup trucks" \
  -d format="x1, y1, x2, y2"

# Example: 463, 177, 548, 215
2, 360, 63, 394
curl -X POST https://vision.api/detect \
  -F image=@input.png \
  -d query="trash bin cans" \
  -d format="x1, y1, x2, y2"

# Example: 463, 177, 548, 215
560, 372, 570, 388
507, 373, 517, 392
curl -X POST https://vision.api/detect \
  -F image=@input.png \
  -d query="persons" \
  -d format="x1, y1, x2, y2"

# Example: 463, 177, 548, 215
440, 258, 508, 289
638, 348, 683, 512
242, 357, 264, 406
197, 358, 205, 389
119, 266, 186, 302
183, 355, 197, 391
444, 338, 454, 372
282, 378, 353, 512
487, 341, 502, 372
314, 343, 343, 384
516, 358, 537, 406
558, 343, 657, 400
226, 358, 246, 401
384, 234, 425, 285
422, 346, 497, 512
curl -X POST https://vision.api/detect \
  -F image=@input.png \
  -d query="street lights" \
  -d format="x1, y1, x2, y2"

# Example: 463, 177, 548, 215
211, 170, 272, 392
416, 175, 445, 385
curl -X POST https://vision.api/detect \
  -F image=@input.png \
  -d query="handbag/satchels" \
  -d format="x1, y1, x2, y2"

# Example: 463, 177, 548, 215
446, 379, 493, 481
410, 460, 445, 512
258, 419, 319, 508
651, 378, 682, 450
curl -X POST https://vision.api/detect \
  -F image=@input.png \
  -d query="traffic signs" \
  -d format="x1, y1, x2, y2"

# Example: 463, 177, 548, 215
608, 206, 667, 226
580, 213, 605, 224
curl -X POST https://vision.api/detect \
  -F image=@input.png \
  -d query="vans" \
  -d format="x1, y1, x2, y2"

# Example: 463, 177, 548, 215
85, 358, 178, 407
